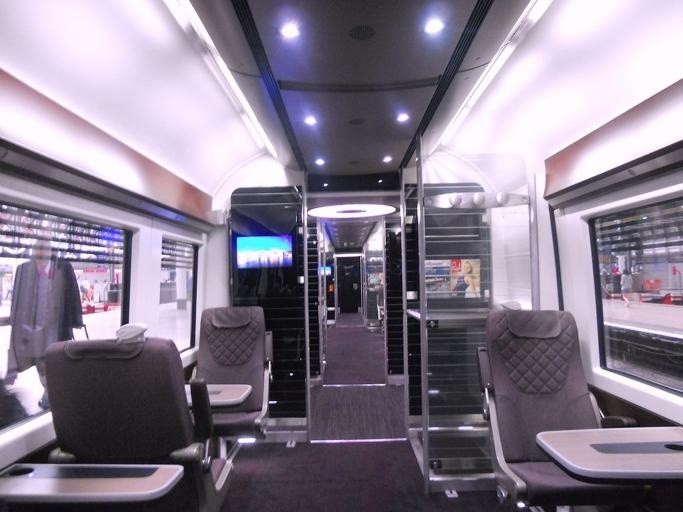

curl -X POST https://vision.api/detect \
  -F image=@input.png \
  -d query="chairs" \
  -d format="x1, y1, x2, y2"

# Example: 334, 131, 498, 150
44, 323, 233, 512
189, 306, 273, 440
476, 309, 637, 512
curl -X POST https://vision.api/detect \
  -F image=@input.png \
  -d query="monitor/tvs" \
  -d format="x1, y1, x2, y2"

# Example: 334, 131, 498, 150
231, 232, 298, 273
425, 255, 484, 302
320, 266, 333, 277
366, 272, 383, 287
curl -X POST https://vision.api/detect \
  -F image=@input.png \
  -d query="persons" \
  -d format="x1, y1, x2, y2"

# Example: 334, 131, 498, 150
620, 269, 633, 303
450, 262, 478, 298
10, 236, 79, 410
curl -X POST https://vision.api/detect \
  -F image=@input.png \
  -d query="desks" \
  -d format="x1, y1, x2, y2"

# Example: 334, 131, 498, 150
184, 383, 253, 410
534, 425, 682, 483
1, 462, 184, 504
